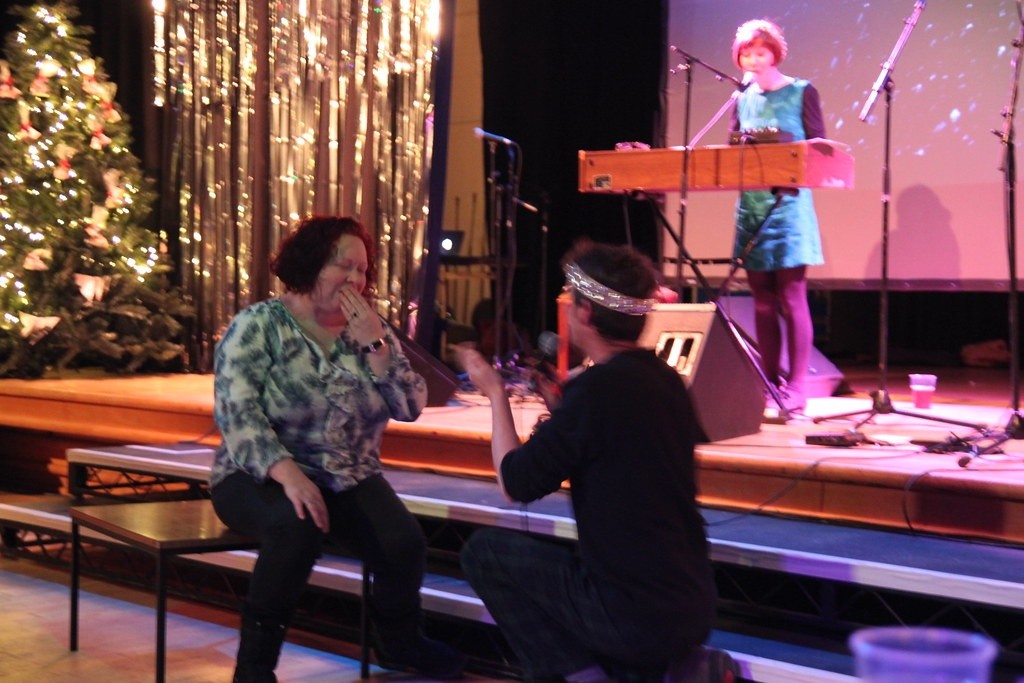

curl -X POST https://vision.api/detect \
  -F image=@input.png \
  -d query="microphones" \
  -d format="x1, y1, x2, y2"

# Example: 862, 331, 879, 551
473, 127, 517, 145
527, 332, 563, 391
732, 71, 758, 98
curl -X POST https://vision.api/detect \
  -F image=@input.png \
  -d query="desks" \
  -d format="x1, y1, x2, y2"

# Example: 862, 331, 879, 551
67, 500, 370, 683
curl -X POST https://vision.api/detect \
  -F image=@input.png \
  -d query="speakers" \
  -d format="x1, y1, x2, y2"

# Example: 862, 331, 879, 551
635, 303, 766, 443
376, 313, 460, 407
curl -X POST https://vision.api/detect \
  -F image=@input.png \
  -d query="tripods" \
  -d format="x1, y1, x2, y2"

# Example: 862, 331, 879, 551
812, 0, 1024, 468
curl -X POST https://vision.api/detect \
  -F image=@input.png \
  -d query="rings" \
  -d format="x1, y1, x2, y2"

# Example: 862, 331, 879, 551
353, 312, 358, 318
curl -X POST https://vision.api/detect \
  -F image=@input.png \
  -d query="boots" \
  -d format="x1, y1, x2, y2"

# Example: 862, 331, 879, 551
233, 617, 288, 683
369, 604, 468, 678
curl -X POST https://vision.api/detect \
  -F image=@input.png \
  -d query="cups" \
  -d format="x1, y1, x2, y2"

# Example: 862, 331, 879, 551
911, 374, 937, 409
848, 626, 1000, 683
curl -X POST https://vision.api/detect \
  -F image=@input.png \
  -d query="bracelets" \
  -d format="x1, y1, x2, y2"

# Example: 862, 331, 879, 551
362, 336, 389, 353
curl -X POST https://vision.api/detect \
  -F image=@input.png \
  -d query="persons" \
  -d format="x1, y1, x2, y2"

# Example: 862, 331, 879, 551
210, 216, 427, 683
728, 20, 825, 418
447, 245, 743, 683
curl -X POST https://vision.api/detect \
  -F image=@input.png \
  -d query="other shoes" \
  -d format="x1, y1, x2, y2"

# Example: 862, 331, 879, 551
764, 386, 807, 414
663, 648, 736, 683
